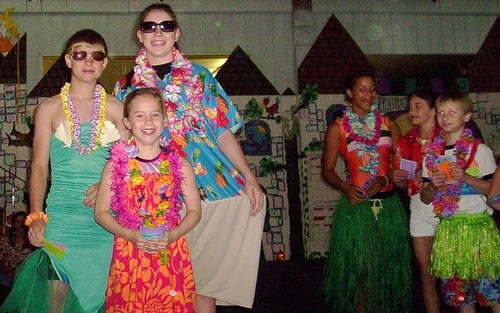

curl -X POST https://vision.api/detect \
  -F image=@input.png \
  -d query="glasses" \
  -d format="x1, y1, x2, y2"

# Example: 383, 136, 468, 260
139, 21, 177, 33
70, 51, 106, 61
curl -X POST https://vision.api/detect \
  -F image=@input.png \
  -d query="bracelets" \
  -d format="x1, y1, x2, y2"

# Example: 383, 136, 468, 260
23, 211, 51, 227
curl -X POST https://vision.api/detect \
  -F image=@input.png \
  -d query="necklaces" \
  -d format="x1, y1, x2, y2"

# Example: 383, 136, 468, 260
56, 81, 107, 154
134, 47, 202, 136
423, 129, 479, 217
339, 104, 385, 177
106, 136, 183, 229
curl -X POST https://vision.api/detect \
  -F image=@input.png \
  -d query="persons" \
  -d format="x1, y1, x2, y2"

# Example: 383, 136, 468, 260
17, 28, 129, 313
93, 86, 203, 313
418, 87, 500, 313
108, 3, 267, 313
386, 90, 457, 313
486, 167, 500, 211
318, 67, 414, 313
